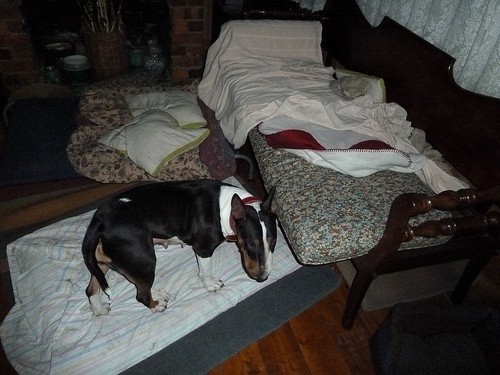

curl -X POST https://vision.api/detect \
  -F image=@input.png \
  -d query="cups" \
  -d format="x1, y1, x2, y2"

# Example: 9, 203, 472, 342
131, 50, 144, 66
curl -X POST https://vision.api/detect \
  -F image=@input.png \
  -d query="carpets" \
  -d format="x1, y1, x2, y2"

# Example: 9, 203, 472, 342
0, 183, 344, 375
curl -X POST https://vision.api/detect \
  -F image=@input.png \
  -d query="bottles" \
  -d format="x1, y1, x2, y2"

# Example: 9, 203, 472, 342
44, 66, 57, 86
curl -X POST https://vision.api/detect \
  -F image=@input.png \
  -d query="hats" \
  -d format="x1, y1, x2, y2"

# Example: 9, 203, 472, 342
330, 75, 370, 99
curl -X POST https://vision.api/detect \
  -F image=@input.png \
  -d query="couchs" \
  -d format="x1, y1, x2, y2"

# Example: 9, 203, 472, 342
222, 0, 500, 328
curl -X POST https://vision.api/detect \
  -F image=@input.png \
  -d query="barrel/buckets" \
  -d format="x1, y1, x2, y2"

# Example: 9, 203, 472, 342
62, 55, 92, 88
142, 45, 172, 80
83, 32, 129, 80
44, 42, 72, 60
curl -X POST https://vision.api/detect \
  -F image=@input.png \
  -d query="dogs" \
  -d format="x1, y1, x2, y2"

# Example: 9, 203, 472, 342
80, 179, 278, 317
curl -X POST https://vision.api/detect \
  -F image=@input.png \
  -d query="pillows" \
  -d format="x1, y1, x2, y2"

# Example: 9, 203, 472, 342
124, 90, 207, 129
97, 109, 211, 177
